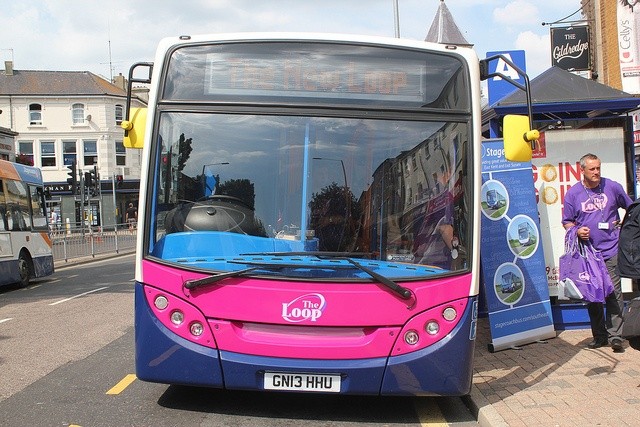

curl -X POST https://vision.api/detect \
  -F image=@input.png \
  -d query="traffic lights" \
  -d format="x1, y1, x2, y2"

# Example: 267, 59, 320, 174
67, 161, 76, 191
117, 175, 123, 184
90, 166, 97, 187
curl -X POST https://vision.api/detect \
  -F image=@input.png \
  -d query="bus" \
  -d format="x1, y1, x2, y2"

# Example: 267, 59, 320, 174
518, 222, 534, 246
122, 33, 539, 396
501, 272, 521, 293
0, 159, 55, 287
486, 190, 505, 209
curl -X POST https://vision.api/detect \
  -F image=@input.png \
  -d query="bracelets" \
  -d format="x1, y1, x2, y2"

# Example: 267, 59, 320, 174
329, 216, 333, 223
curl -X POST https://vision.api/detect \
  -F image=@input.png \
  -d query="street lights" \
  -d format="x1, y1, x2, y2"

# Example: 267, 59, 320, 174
313, 157, 349, 251
202, 162, 229, 195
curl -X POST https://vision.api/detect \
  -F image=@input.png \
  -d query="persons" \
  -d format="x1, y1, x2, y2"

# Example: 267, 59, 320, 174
410, 162, 454, 270
561, 153, 634, 351
125, 202, 137, 235
317, 173, 347, 252
50, 209, 57, 237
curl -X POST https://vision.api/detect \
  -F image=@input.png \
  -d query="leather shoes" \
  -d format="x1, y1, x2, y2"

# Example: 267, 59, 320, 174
589, 340, 608, 349
611, 339, 623, 352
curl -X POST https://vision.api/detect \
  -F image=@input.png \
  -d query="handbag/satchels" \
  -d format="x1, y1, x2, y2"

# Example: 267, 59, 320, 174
558, 226, 615, 303
